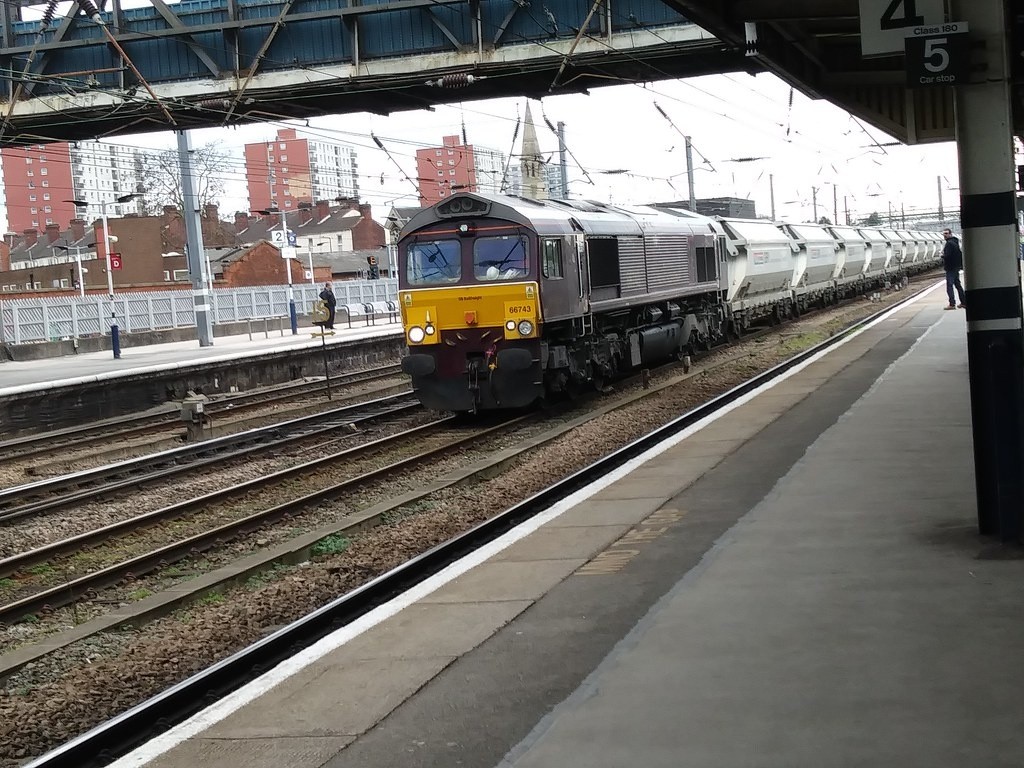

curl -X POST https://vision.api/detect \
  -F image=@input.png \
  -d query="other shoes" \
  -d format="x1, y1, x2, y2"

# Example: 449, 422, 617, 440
943, 305, 955, 310
957, 304, 965, 308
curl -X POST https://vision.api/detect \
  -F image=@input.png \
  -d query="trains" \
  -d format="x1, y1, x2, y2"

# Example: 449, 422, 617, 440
396, 185, 964, 417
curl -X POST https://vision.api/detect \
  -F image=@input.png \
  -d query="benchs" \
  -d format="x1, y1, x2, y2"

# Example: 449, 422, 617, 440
342, 301, 401, 328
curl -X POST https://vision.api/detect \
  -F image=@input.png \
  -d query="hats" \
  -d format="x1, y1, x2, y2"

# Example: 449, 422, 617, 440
486, 266, 499, 280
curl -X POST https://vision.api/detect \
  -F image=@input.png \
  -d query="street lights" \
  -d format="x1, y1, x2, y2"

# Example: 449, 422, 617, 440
374, 244, 400, 278
294, 242, 325, 313
249, 207, 315, 335
60, 193, 143, 361
53, 242, 101, 339
384, 215, 418, 285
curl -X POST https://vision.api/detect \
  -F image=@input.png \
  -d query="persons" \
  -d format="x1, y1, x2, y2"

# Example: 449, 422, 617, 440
318, 281, 337, 330
941, 228, 969, 310
